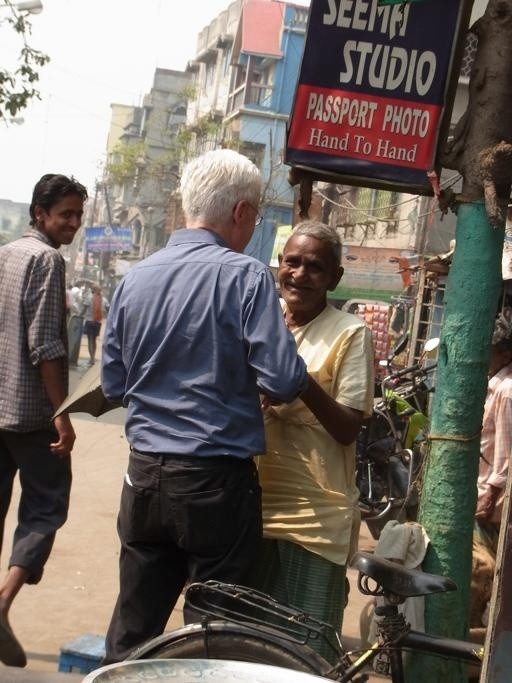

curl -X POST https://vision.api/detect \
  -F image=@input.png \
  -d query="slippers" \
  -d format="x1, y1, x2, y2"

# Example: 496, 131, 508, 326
1, 618, 27, 668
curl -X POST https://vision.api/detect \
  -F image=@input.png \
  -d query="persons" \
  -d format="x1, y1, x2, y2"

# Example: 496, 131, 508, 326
253, 221, 375, 667
474, 307, 512, 640
0, 174, 88, 667
101, 149, 305, 665
66, 278, 109, 369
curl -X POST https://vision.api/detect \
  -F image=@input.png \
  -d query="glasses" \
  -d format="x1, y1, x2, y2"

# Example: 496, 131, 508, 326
246, 201, 264, 226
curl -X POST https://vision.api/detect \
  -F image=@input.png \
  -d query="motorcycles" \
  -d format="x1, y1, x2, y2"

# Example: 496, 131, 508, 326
358, 330, 448, 542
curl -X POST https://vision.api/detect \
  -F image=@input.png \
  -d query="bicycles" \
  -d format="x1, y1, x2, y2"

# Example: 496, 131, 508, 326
116, 551, 485, 681
